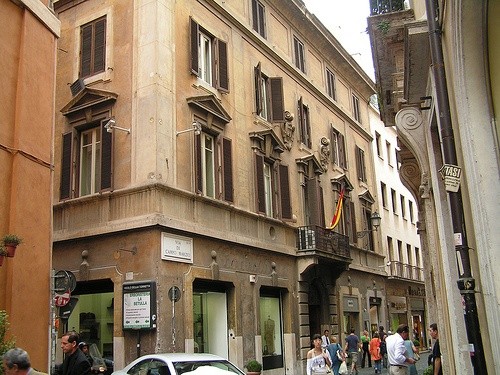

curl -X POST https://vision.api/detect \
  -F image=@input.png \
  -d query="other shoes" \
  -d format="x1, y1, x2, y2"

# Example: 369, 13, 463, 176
375, 369, 378, 373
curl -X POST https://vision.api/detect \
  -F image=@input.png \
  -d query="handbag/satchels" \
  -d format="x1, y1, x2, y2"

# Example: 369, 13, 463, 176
324, 349, 334, 375
338, 361, 348, 375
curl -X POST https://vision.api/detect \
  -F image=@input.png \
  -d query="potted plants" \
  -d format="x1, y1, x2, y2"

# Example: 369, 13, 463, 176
246, 360, 263, 375
0, 234, 24, 266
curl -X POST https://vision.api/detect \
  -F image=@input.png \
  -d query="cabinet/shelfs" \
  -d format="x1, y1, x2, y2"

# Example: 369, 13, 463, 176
80, 312, 100, 343
106, 306, 113, 325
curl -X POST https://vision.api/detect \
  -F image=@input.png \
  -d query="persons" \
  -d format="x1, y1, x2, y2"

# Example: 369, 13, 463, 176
327, 337, 344, 375
369, 332, 382, 375
379, 326, 387, 368
2, 348, 49, 375
429, 324, 443, 375
264, 314, 276, 354
404, 337, 418, 375
321, 330, 330, 353
55, 331, 92, 375
344, 328, 361, 375
79, 342, 104, 375
385, 324, 416, 375
307, 334, 333, 375
361, 330, 372, 368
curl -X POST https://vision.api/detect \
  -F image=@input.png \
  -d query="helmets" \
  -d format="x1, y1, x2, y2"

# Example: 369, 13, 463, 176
78, 342, 88, 350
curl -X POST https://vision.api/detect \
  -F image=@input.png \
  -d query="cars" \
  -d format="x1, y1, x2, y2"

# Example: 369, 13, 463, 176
110, 352, 248, 375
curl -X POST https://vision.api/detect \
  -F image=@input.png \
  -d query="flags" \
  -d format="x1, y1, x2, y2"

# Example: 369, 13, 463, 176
326, 187, 345, 230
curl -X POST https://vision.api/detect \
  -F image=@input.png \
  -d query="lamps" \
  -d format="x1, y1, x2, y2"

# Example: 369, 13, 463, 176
356, 211, 381, 238
